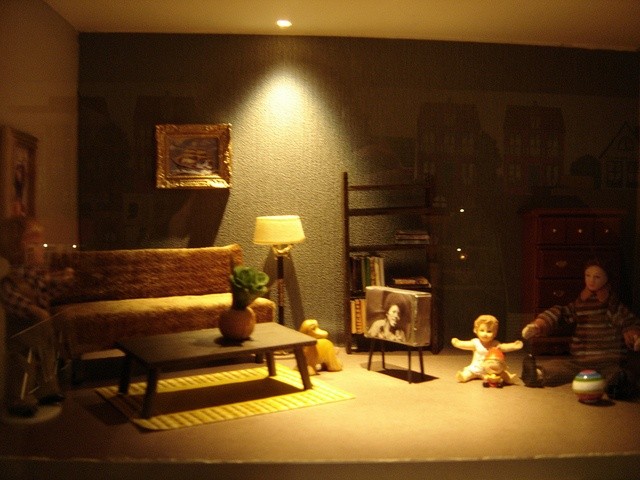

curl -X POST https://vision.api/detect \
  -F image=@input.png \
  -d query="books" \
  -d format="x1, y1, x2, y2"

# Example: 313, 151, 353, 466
348, 254, 386, 294
351, 299, 366, 335
390, 275, 429, 285
394, 228, 430, 245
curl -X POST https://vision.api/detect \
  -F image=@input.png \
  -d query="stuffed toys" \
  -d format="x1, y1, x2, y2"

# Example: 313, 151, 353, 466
482, 346, 506, 388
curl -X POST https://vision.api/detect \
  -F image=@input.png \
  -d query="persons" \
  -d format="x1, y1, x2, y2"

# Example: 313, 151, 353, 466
366, 292, 410, 343
521, 261, 639, 388
0, 209, 74, 417
451, 314, 524, 384
